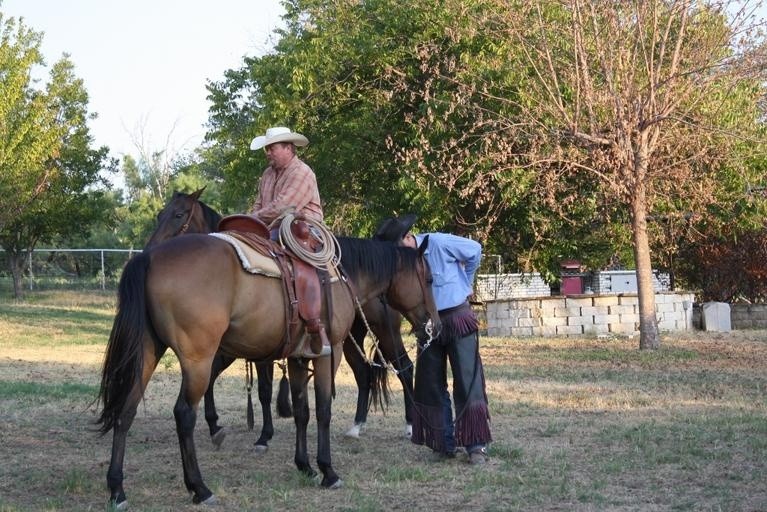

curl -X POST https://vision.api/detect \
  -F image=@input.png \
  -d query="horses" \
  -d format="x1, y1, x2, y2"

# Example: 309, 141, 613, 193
143, 186, 414, 453
87, 228, 442, 509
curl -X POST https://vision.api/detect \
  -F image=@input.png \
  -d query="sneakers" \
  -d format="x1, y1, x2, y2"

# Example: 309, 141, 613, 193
310, 330, 324, 353
470, 446, 490, 464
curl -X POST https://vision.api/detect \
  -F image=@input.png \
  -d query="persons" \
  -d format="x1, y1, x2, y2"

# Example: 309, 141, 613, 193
243, 126, 325, 355
370, 217, 494, 466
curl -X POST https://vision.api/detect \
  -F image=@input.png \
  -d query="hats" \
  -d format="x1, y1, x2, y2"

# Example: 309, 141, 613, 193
372, 213, 418, 242
249, 127, 310, 151
